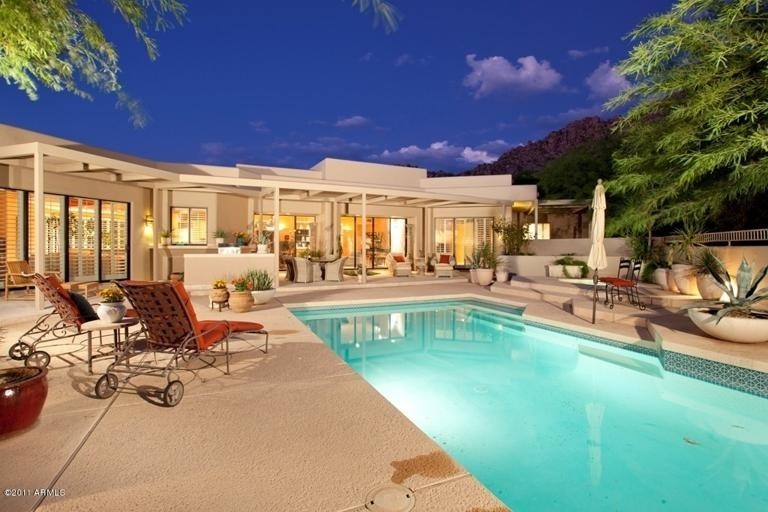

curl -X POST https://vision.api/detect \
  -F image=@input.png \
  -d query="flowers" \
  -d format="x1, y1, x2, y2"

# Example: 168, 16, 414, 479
210, 277, 228, 290
234, 276, 252, 291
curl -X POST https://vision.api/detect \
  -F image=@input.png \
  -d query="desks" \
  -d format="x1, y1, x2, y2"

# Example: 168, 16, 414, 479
308, 258, 336, 282
80, 321, 138, 372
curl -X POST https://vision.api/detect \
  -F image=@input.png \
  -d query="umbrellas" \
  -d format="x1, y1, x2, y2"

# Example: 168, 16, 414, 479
585, 178, 610, 324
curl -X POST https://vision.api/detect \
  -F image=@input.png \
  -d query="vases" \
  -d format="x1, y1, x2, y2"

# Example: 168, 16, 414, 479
212, 291, 230, 304
0, 367, 49, 433
230, 292, 254, 312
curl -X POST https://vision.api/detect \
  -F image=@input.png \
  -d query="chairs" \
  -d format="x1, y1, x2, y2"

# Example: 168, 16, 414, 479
599, 258, 630, 307
606, 259, 648, 310
4, 260, 62, 299
430, 252, 456, 279
10, 271, 118, 372
290, 256, 312, 282
324, 257, 349, 284
93, 277, 269, 408
388, 253, 411, 278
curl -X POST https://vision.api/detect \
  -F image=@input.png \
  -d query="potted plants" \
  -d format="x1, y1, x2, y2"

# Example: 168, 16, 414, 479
212, 229, 273, 254
688, 246, 768, 355
242, 270, 277, 305
160, 224, 175, 245
668, 224, 701, 291
467, 241, 495, 284
98, 285, 129, 323
696, 247, 733, 299
